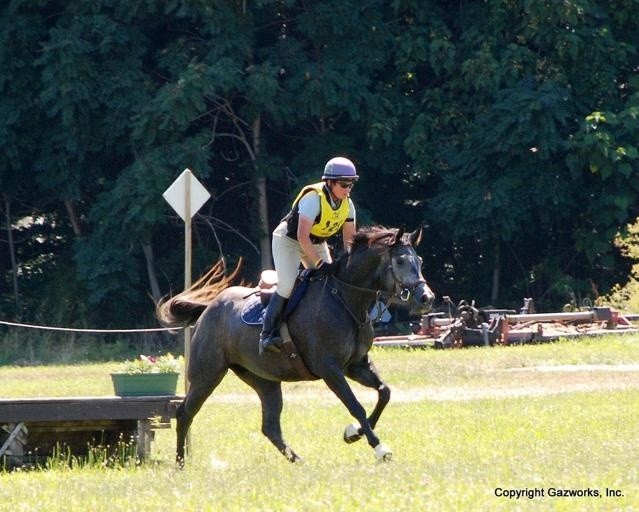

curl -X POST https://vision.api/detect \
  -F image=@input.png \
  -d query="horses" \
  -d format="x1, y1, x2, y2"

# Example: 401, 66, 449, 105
147, 225, 435, 470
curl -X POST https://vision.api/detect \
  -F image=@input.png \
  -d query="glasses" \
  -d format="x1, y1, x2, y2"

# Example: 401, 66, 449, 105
335, 181, 353, 189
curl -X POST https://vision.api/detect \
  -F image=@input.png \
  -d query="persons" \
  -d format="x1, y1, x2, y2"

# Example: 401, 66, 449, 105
460, 310, 488, 329
521, 297, 536, 314
259, 157, 359, 347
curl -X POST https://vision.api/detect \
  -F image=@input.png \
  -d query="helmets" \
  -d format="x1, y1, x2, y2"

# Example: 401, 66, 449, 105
321, 156, 360, 181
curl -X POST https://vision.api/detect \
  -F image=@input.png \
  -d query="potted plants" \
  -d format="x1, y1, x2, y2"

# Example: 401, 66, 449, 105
108, 353, 185, 395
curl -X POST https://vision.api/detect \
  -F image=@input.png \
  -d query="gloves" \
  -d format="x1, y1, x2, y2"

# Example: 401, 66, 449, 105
315, 258, 333, 273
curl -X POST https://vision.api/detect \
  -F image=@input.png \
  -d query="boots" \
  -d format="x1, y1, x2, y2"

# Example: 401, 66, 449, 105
259, 291, 290, 354
